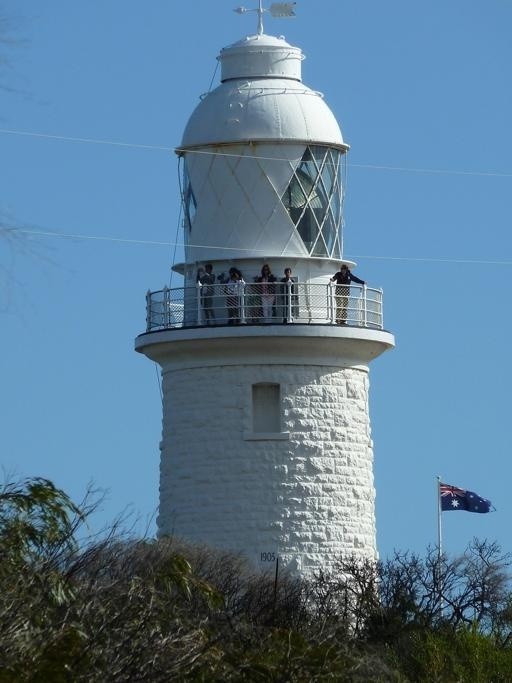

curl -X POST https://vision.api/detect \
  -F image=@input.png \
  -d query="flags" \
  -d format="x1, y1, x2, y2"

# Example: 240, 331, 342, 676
439, 483, 497, 513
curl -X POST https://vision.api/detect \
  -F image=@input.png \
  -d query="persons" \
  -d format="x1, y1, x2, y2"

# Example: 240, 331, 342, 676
280, 267, 294, 323
330, 265, 366, 324
196, 264, 215, 325
254, 264, 277, 322
219, 267, 247, 325
